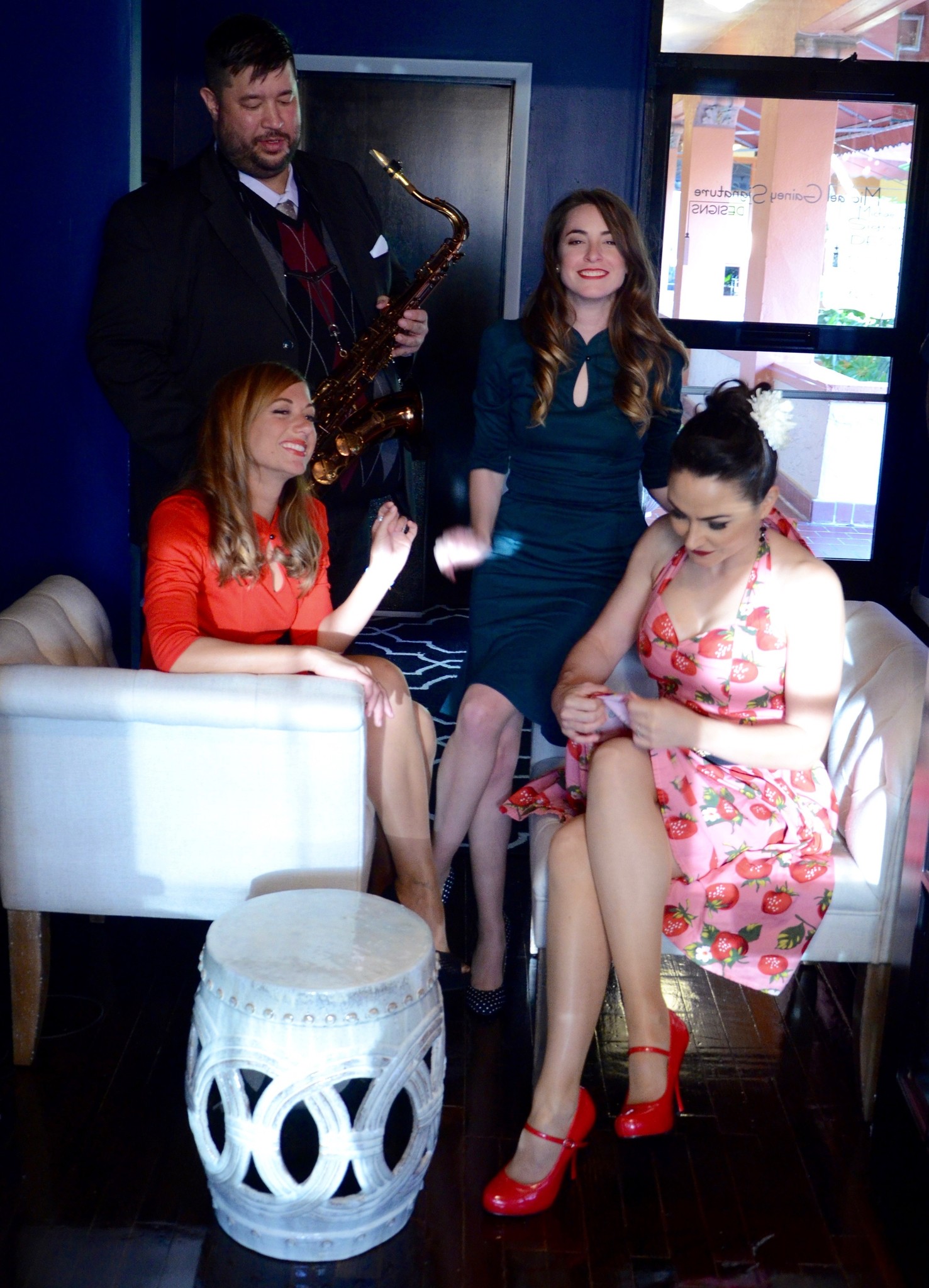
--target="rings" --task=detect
[377,516,383,521]
[402,528,405,530]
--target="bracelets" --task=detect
[365,567,395,590]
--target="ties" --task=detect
[280,200,296,220]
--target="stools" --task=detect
[183,887,449,1263]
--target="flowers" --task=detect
[745,387,796,451]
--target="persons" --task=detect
[483,374,846,1220]
[428,189,686,1027]
[91,13,431,672]
[144,359,470,974]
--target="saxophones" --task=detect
[305,145,471,487]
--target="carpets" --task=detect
[352,605,533,850]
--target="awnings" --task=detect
[671,0,929,156]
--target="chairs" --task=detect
[528,594,929,1120]
[0,570,379,1067]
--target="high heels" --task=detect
[466,916,509,1016]
[442,867,456,906]
[615,1010,690,1139]
[484,1085,596,1215]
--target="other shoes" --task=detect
[438,950,459,996]
